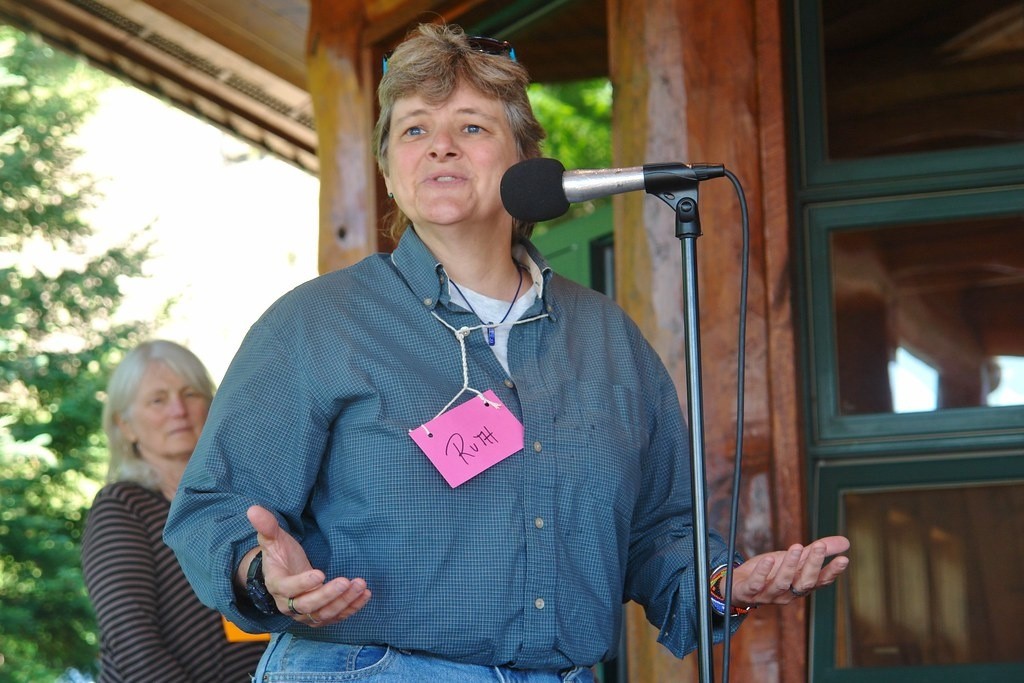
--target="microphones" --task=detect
[499,156,725,224]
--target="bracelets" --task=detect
[707,562,755,618]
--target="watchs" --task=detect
[246,550,279,618]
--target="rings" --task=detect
[288,596,303,615]
[789,584,808,596]
[307,613,319,623]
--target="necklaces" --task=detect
[449,259,523,346]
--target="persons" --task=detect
[161,26,851,683]
[79,339,269,683]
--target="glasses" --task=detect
[382,35,517,77]
